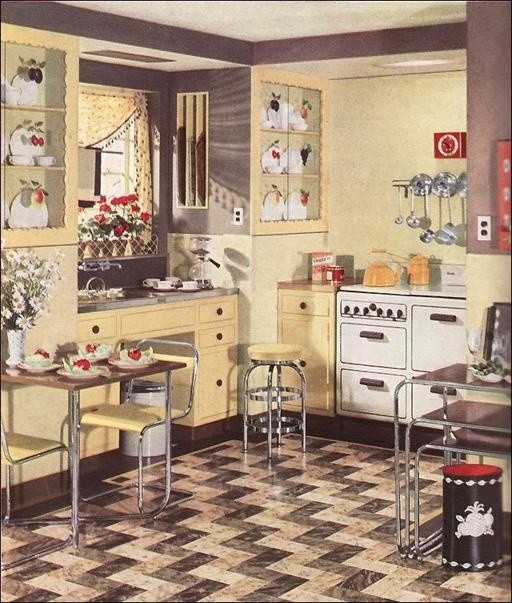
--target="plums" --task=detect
[28,68,42,84]
[270,100,280,112]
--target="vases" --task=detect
[3,327,25,370]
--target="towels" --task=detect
[11,341,154,378]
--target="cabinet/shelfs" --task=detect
[168,294,240,440]
[74,312,121,460]
[275,280,340,417]
[120,304,193,333]
[166,67,329,236]
[1,22,80,247]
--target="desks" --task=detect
[389,361,512,563]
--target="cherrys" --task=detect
[272,151,280,159]
[31,135,44,146]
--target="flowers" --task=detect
[78,191,151,256]
[1,238,62,330]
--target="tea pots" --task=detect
[363,248,404,286]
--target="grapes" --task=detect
[300,149,308,166]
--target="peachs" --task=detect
[273,190,280,206]
[30,189,46,208]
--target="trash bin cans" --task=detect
[120,380,168,458]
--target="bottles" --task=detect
[408,252,429,285]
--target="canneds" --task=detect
[324,264,344,284]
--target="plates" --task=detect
[67,353,108,362]
[261,101,308,222]
[107,358,159,368]
[7,71,49,229]
[17,361,62,373]
[56,368,100,379]
[472,372,503,383]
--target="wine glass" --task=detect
[465,326,481,371]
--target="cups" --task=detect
[79,288,122,301]
[141,275,201,292]
[36,156,56,166]
[325,266,344,283]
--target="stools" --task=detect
[242,341,310,464]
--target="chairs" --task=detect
[77,337,201,519]
[0,407,79,572]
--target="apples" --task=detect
[299,194,308,207]
[74,358,90,370]
[86,344,96,353]
[128,348,141,360]
[33,349,49,359]
[299,107,308,118]
